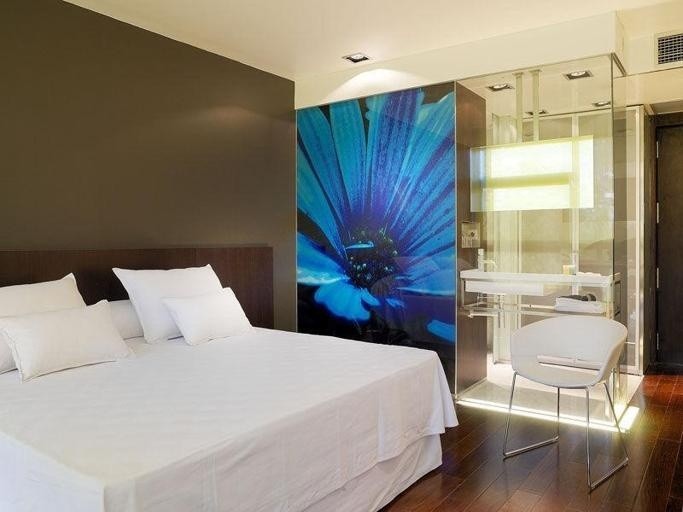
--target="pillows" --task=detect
[0,269,87,377]
[111,264,222,344]
[0,298,131,382]
[108,300,145,342]
[158,286,253,346]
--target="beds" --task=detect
[0,245,460,512]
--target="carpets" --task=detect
[462,381,615,424]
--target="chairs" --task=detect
[497,315,631,494]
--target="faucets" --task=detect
[477,248,499,272]
